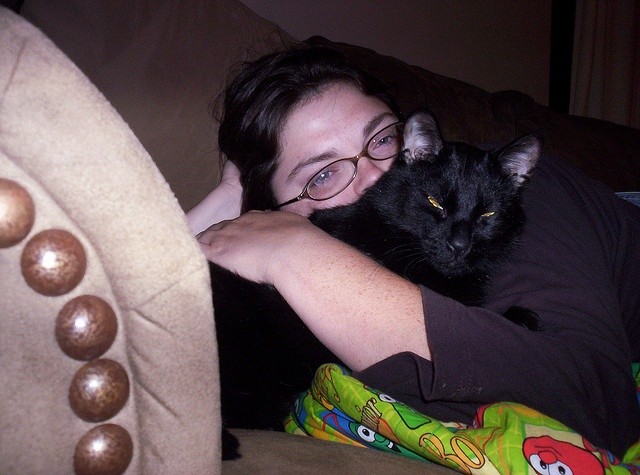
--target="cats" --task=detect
[207,111,542,461]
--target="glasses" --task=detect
[276,120,409,208]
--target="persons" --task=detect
[183,45,640,463]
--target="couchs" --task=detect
[2,2,639,473]
